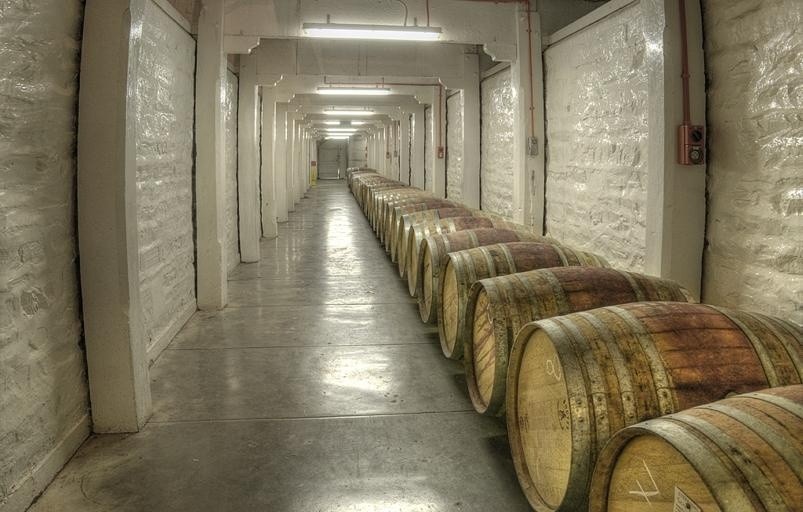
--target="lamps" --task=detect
[320,119,365,140]
[321,105,378,116]
[300,22,443,41]
[316,85,392,97]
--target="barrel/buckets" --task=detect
[390,201,457,263]
[436,242,615,361]
[417,224,564,327]
[386,197,444,254]
[399,207,488,280]
[346,166,433,243]
[504,301,803,512]
[464,266,699,415]
[405,213,511,298]
[587,384,801,510]
